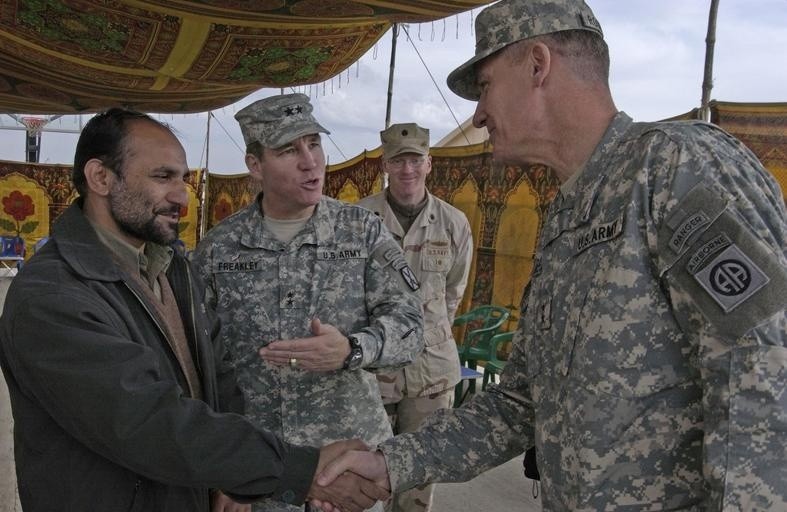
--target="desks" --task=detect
[0,256,24,277]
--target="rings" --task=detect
[288,357,297,367]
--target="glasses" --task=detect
[385,158,425,167]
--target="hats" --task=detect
[380,123,429,159]
[234,93,331,150]
[447,0,603,100]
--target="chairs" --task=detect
[0,237,51,270]
[453,305,515,407]
[173,239,195,259]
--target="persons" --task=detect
[186,92,425,510]
[0,107,391,511]
[349,122,475,511]
[299,1,784,510]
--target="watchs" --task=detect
[343,335,363,372]
